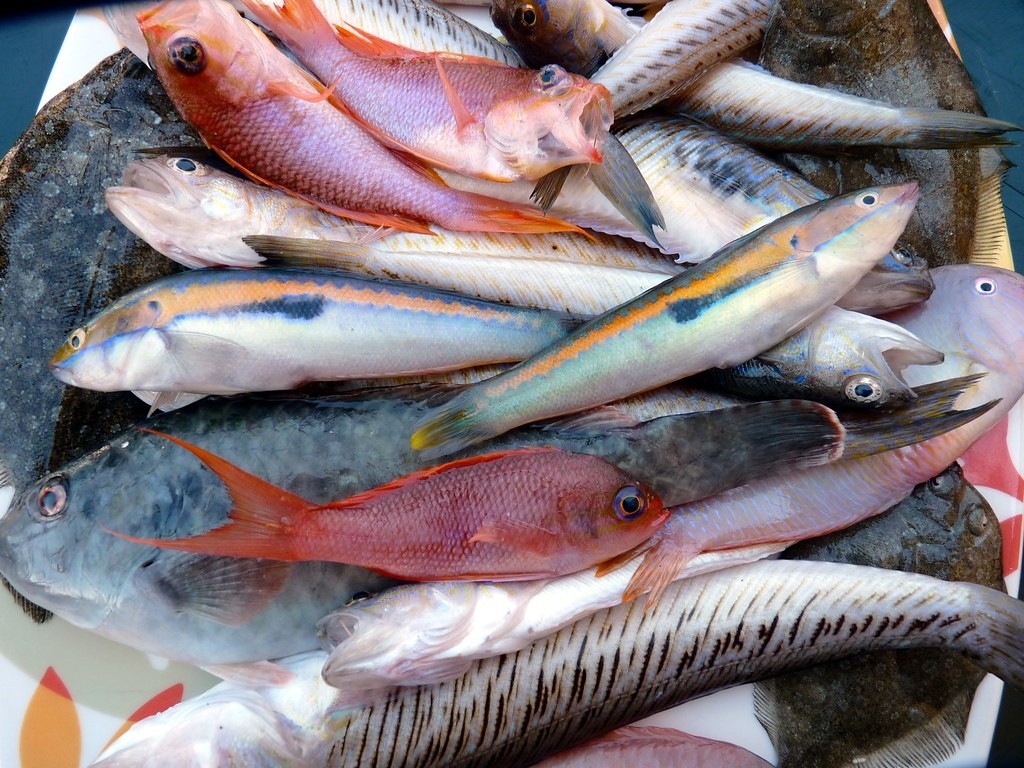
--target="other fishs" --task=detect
[0,2,1024,768]
